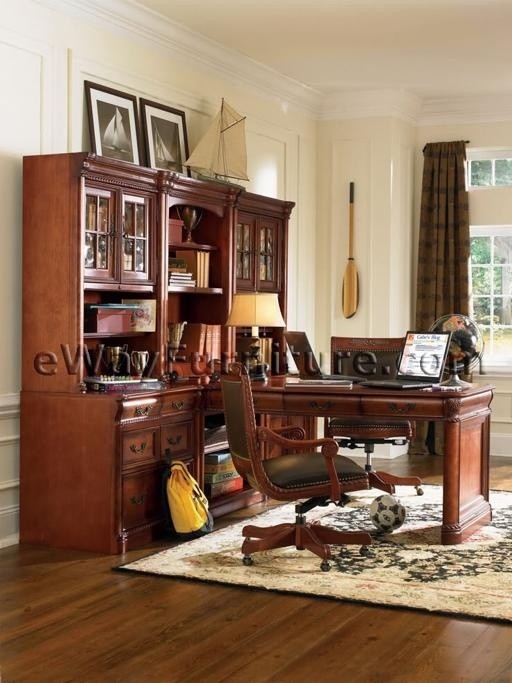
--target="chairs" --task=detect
[212,360,374,572]
[327,334,427,495]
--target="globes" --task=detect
[428,313,485,391]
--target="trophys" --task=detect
[176,204,204,244]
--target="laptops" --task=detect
[359,330,453,389]
[283,331,368,384]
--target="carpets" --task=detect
[113,484,512,623]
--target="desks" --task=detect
[202,375,498,542]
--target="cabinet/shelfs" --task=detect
[19,375,317,553]
[22,149,295,393]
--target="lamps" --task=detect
[226,290,286,375]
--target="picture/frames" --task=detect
[138,93,193,178]
[82,79,146,167]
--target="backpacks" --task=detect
[161,461,214,537]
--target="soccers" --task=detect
[369,495,406,532]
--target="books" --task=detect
[168,257,196,287]
[205,452,243,498]
[177,250,209,287]
[240,337,272,371]
[285,377,351,388]
[123,300,156,332]
[183,322,238,374]
[91,302,143,309]
